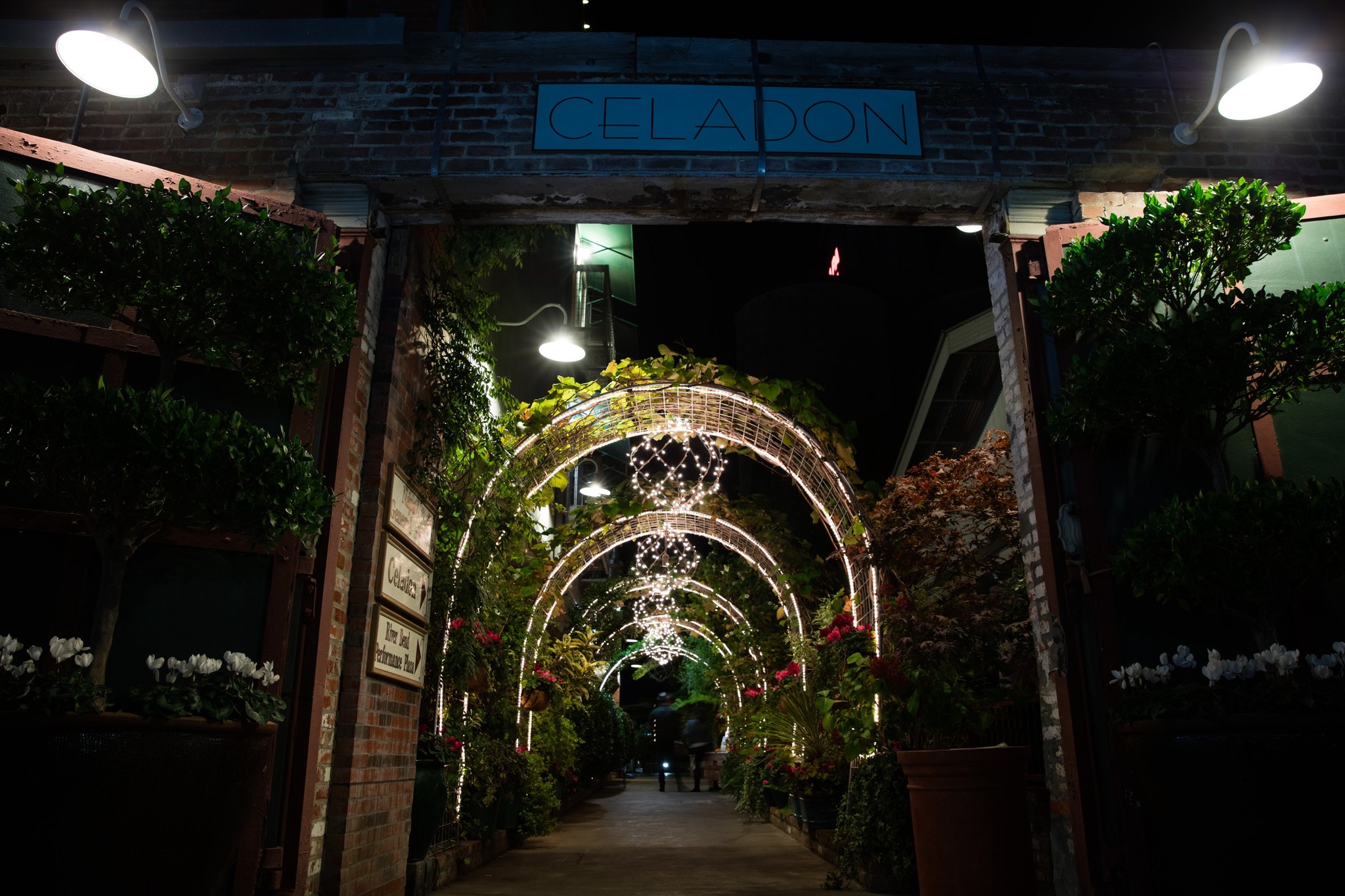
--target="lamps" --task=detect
[622,436,730,659]
[567,455,611,496]
[55,2,202,133]
[489,297,591,368]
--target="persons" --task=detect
[682,711,722,794]
[648,691,692,791]
